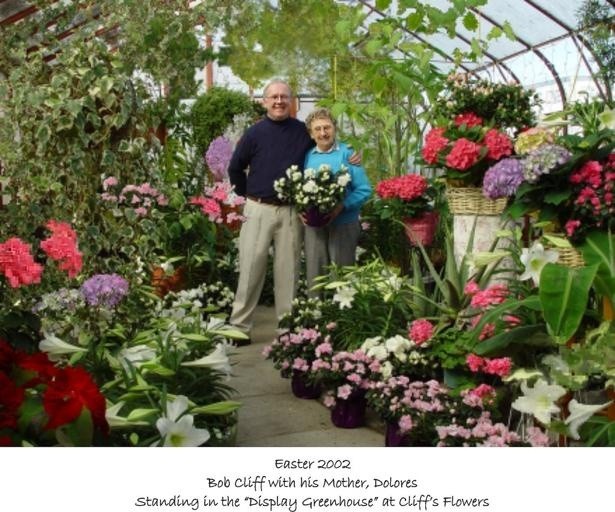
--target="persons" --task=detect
[222,76,363,348]
[297,107,374,306]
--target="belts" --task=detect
[245,195,280,206]
